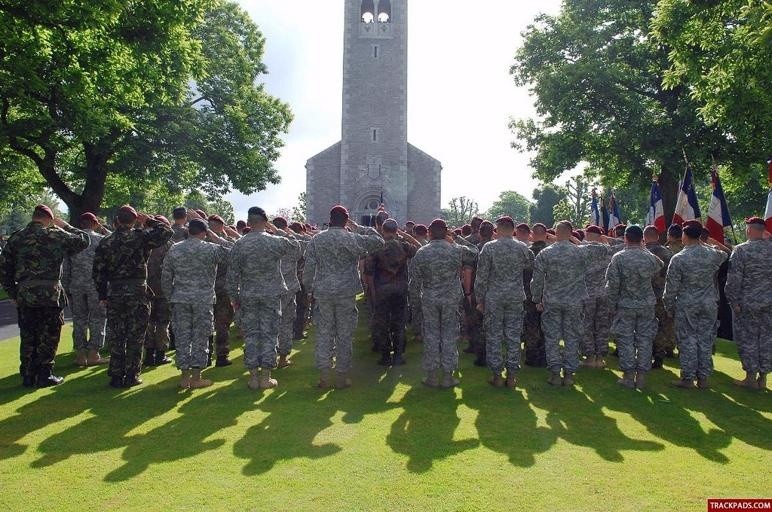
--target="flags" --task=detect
[762,159,772,241]
[607,192,622,232]
[671,163,703,226]
[590,192,600,226]
[645,182,667,235]
[598,197,609,229]
[704,165,732,245]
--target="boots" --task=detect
[637,371,645,388]
[319,368,331,387]
[261,367,277,389]
[336,371,352,389]
[179,370,191,389]
[377,353,392,365]
[88,351,108,366]
[248,367,259,389]
[74,351,87,366]
[735,371,759,389]
[192,369,211,389]
[758,373,767,390]
[393,352,404,365]
[618,370,635,388]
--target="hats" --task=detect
[461,225,471,231]
[414,224,428,231]
[208,214,225,224]
[291,221,302,230]
[454,229,462,233]
[330,206,349,216]
[154,214,170,225]
[120,205,138,217]
[273,217,288,227]
[195,210,209,220]
[764,217,772,234]
[572,231,583,242]
[249,207,267,220]
[585,226,601,233]
[682,220,701,227]
[430,219,447,228]
[174,208,186,213]
[745,217,766,224]
[479,220,492,228]
[578,230,585,238]
[406,221,414,226]
[237,220,245,227]
[376,212,389,219]
[80,213,98,224]
[35,204,53,220]
[190,218,208,231]
[518,223,530,232]
[612,224,627,232]
[496,216,514,227]
[546,230,556,235]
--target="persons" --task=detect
[235,220,247,236]
[603,225,666,390]
[567,231,582,246]
[301,204,386,391]
[223,206,303,391]
[607,229,616,238]
[723,237,732,251]
[578,224,626,369]
[160,217,236,390]
[463,216,485,354]
[363,217,424,367]
[401,221,415,236]
[522,222,556,369]
[723,216,772,391]
[407,217,480,388]
[544,229,556,244]
[170,206,210,244]
[358,211,412,352]
[462,219,498,368]
[91,204,175,388]
[303,223,313,237]
[514,223,534,343]
[290,221,316,340]
[699,226,732,375]
[61,211,114,367]
[576,228,585,239]
[661,218,729,390]
[222,226,238,240]
[663,223,686,359]
[643,224,674,369]
[474,215,536,389]
[143,214,178,368]
[270,216,312,367]
[204,213,244,367]
[0,202,92,388]
[449,228,463,242]
[530,220,608,388]
[460,224,472,238]
[407,223,429,344]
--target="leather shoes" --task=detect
[143,349,155,368]
[156,351,173,366]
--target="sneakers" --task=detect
[421,376,439,388]
[563,376,575,385]
[609,347,618,356]
[39,374,63,387]
[474,357,487,367]
[462,344,477,354]
[652,358,662,368]
[539,357,546,367]
[524,359,537,368]
[111,376,124,388]
[294,331,309,340]
[216,356,231,367]
[23,376,37,388]
[673,379,694,387]
[666,349,674,357]
[549,375,562,387]
[598,356,607,367]
[207,357,212,367]
[697,377,708,388]
[506,376,519,389]
[442,376,461,388]
[278,354,293,367]
[583,356,596,366]
[125,376,142,388]
[494,375,505,388]
